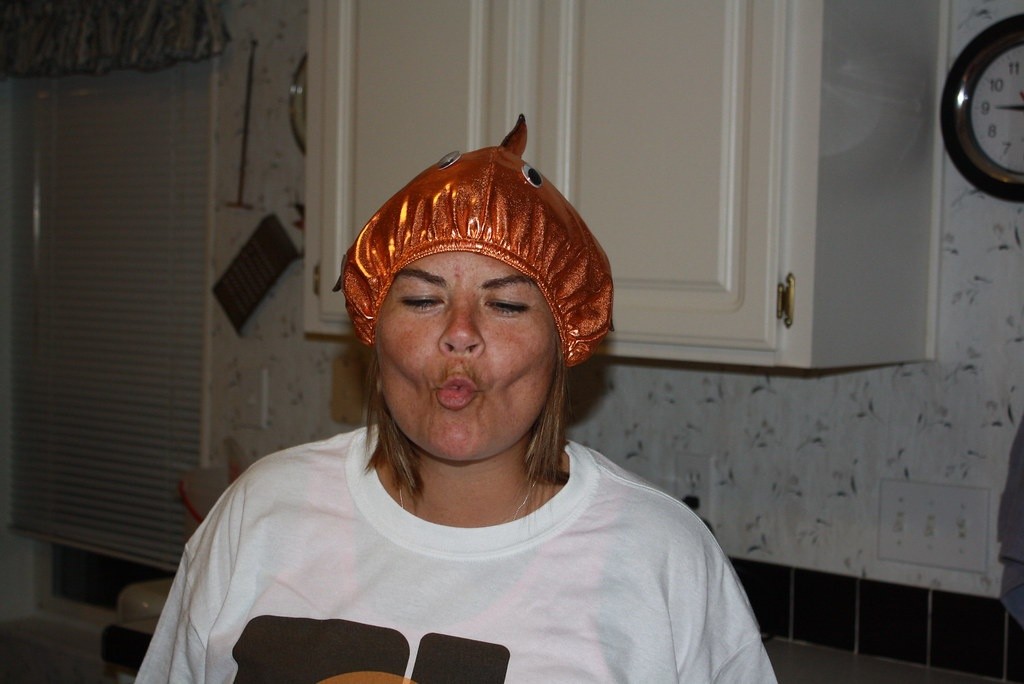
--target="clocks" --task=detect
[939,10,1024,206]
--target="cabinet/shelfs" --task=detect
[302,3,943,372]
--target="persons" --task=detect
[131,113,785,684]
[997,403,1024,642]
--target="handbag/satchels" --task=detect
[211,214,303,336]
